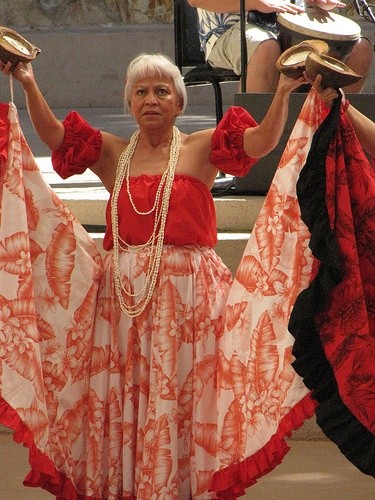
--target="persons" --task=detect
[302,70,375,166]
[187,0,373,94]
[0,53,335,500]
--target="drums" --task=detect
[277,7,361,64]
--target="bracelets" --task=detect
[344,99,350,111]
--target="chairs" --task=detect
[173,0,242,179]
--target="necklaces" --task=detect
[109,124,182,319]
